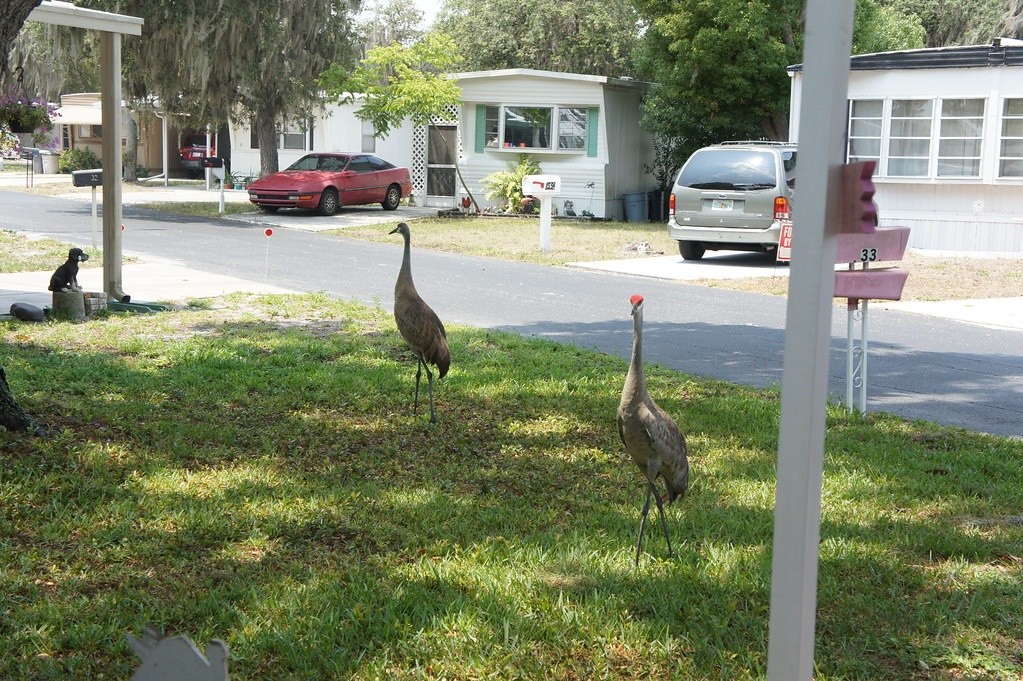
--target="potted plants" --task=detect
[224,168,253,190]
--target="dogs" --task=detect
[48,247,90,292]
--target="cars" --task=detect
[248,151,410,217]
[667,140,800,265]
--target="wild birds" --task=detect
[615,294,689,571]
[388,222,450,425]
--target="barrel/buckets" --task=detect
[623,192,649,223]
[650,191,669,222]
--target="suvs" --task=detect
[178,128,231,177]
